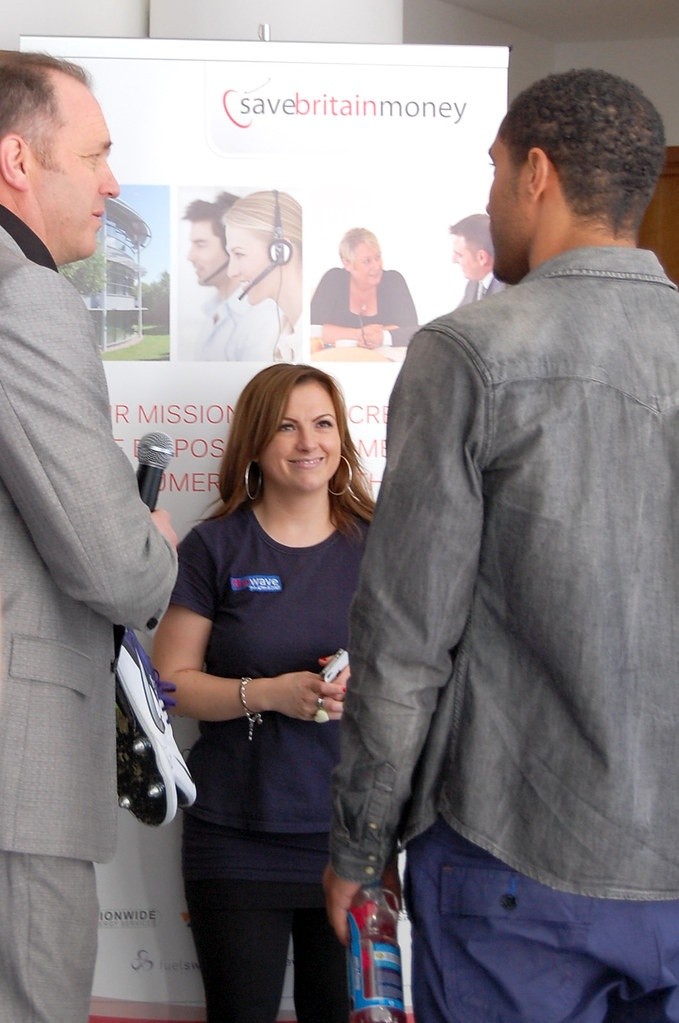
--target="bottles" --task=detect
[345,877,408,1023]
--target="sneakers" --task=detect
[110,621,196,827]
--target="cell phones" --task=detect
[321,649,349,683]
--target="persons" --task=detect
[0,50,183,1023]
[319,66,679,1023]
[448,214,508,308]
[176,191,302,363]
[311,228,418,351]
[155,364,404,1022]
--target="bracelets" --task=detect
[240,678,263,741]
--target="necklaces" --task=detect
[362,305,367,310]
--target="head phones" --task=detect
[268,190,293,264]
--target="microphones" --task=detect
[238,262,279,301]
[358,305,369,346]
[204,261,229,282]
[136,433,175,512]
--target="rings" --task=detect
[313,707,329,723]
[317,696,324,708]
